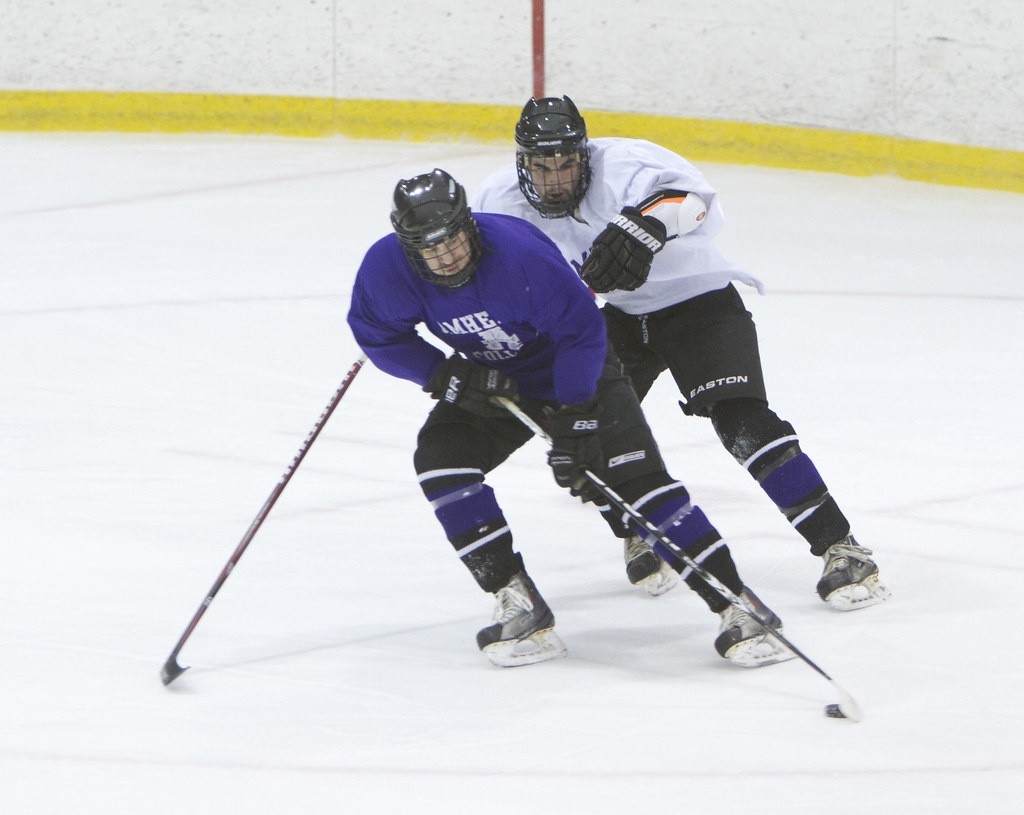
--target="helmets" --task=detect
[390,168,482,291]
[515,95,592,219]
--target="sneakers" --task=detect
[477,552,568,667]
[624,535,679,596]
[714,585,804,668]
[816,535,892,611]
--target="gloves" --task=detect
[547,395,605,504]
[423,351,520,417]
[581,206,666,293]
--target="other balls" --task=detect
[825,702,847,719]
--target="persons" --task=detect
[345,168,799,668]
[471,95,894,612]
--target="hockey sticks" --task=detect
[158,345,369,684]
[494,398,869,725]
